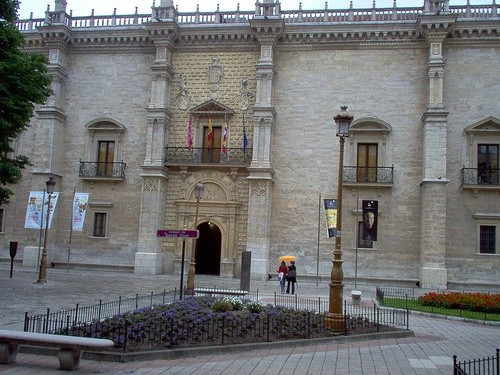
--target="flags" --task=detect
[186,116,193,150]
[207,118,213,148]
[72,193,90,231]
[243,125,248,148]
[24,191,59,229]
[221,122,227,155]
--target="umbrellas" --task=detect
[278,255,296,264]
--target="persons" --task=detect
[286,261,296,294]
[276,261,287,294]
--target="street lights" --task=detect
[36,177,56,283]
[182,180,205,295]
[323,105,355,333]
